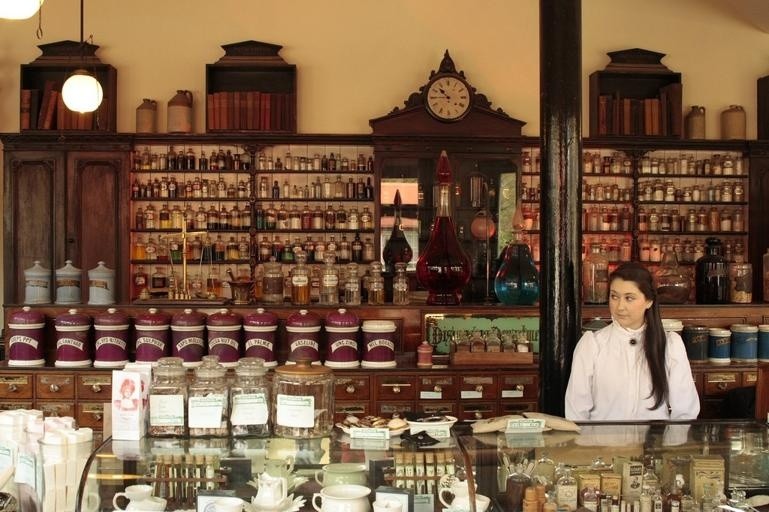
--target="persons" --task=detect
[565,262,700,448]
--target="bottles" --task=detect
[256,273,264,302]
[682,238,693,262]
[650,239,662,263]
[151,453,215,503]
[486,331,502,352]
[383,189,413,303]
[638,235,651,261]
[620,239,632,262]
[653,247,690,304]
[368,263,384,305]
[455,331,470,354]
[310,267,319,302]
[763,248,769,305]
[472,331,486,353]
[673,240,683,264]
[338,268,345,300]
[515,334,532,352]
[263,256,285,304]
[583,244,609,305]
[132,234,251,306]
[726,239,732,263]
[503,451,757,512]
[394,451,457,494]
[345,264,360,305]
[291,252,310,304]
[695,238,726,304]
[320,253,341,304]
[522,146,748,234]
[283,270,293,299]
[259,235,374,260]
[609,238,621,261]
[495,199,542,306]
[601,239,608,261]
[392,263,409,304]
[502,334,516,352]
[729,256,751,304]
[416,150,474,306]
[735,239,744,262]
[694,240,704,262]
[583,240,589,259]
[130,142,373,231]
[362,271,370,302]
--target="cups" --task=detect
[216,498,244,511]
[311,484,371,512]
[313,463,366,486]
[373,500,402,512]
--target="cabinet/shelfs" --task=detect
[582,315,767,403]
[205,38,298,136]
[581,144,753,306]
[32,139,130,306]
[130,140,375,307]
[332,372,540,430]
[369,50,527,302]
[0,372,109,431]
[586,48,683,140]
[19,39,117,132]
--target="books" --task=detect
[208,90,293,131]
[598,83,682,137]
[21,81,110,132]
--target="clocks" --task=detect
[422,70,472,121]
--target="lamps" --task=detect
[62,0,103,113]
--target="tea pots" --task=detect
[112,485,167,511]
[439,483,491,512]
[266,455,294,485]
[253,472,286,507]
